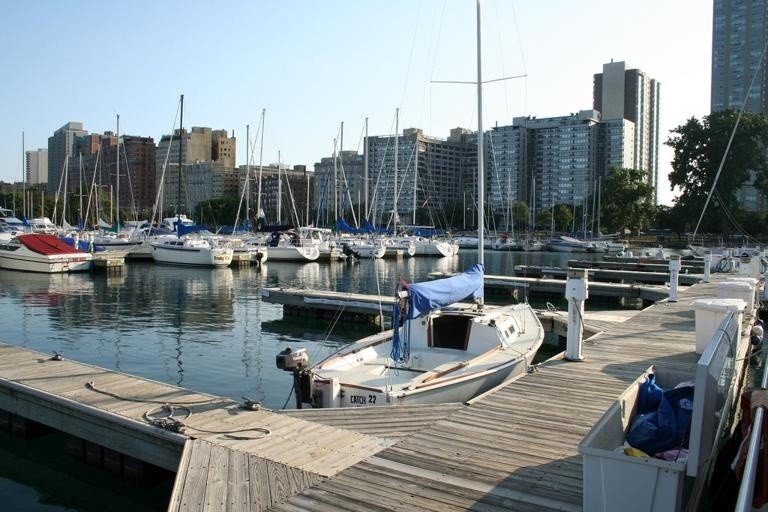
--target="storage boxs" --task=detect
[576,312,739,512]
[692,276,757,356]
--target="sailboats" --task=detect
[274,1,545,408]
[687,42,768,258]
[0,94,630,274]
